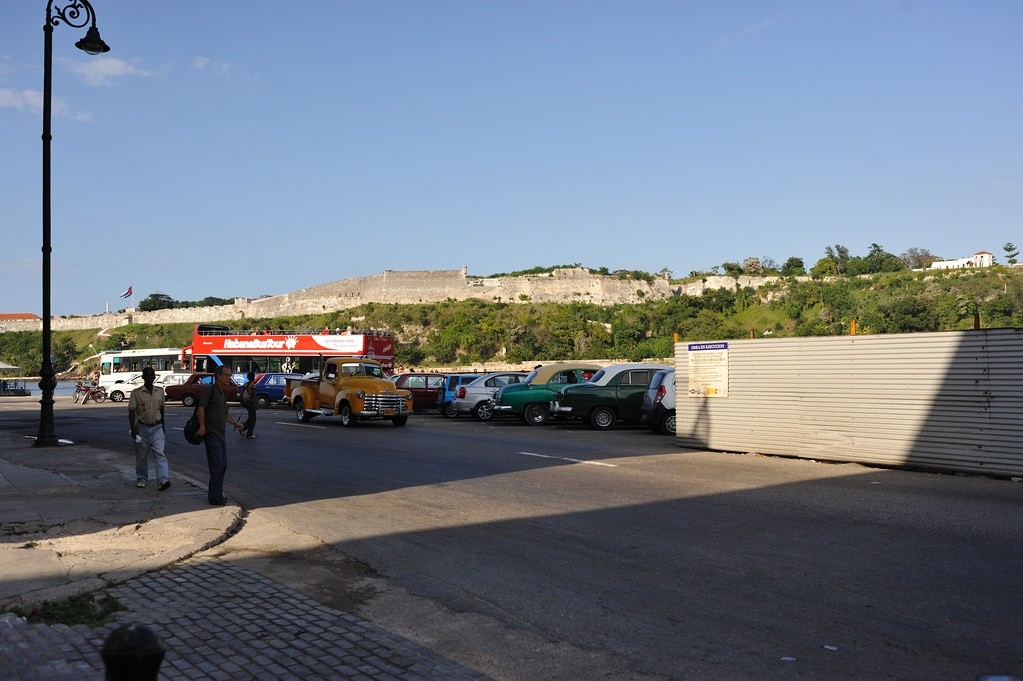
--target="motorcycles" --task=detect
[71,382,107,404]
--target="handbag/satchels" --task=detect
[184,414,205,445]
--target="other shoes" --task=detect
[240,431,245,437]
[247,434,257,438]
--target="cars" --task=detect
[550,363,671,429]
[165,374,243,407]
[488,363,605,426]
[641,369,676,435]
[451,372,528,423]
[237,373,304,408]
[436,374,507,418]
[389,374,446,413]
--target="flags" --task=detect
[120,286,133,300]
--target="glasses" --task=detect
[222,374,232,376]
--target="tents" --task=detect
[0,361,22,377]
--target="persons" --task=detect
[239,372,260,439]
[195,365,244,504]
[0,379,18,392]
[320,325,361,335]
[255,325,284,335]
[581,370,592,382]
[128,367,171,491]
[93,372,99,388]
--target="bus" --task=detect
[97,346,205,387]
[182,324,395,383]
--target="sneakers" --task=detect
[158,480,171,491]
[137,479,147,487]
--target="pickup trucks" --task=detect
[281,356,413,427]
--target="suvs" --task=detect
[104,372,176,400]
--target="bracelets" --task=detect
[233,421,238,425]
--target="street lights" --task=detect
[32,0,113,446]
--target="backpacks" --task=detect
[240,387,250,405]
